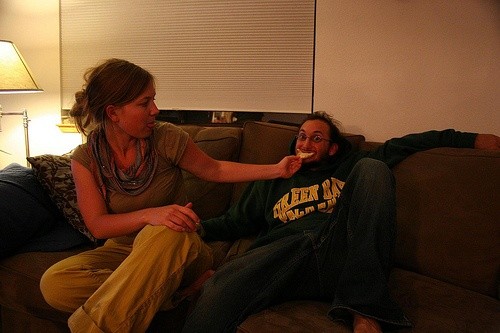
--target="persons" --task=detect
[152,110,499,332]
[35,58,304,333]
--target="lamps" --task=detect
[0,39,45,169]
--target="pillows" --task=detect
[25,152,105,245]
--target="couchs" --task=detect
[0,121,498,333]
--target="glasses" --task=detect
[295,133,331,143]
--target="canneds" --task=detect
[212,111,233,123]
